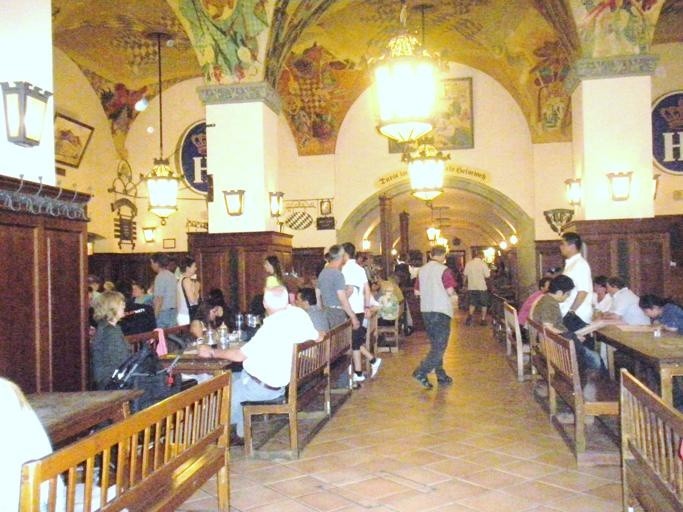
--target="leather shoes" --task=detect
[229,431,246,446]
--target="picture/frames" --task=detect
[53,109,95,170]
[387,77,475,153]
[162,238,176,249]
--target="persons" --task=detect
[462,251,490,326]
[600,277,650,326]
[411,245,456,389]
[510,276,552,346]
[199,285,325,449]
[638,295,682,333]
[88,251,230,467]
[317,242,414,388]
[529,274,598,388]
[0,377,130,511]
[264,255,330,333]
[592,275,607,303]
[556,230,595,387]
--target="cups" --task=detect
[204,311,257,350]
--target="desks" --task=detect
[20,387,144,449]
[588,317,681,407]
[155,327,262,397]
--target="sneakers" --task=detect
[463,316,470,325]
[480,318,488,325]
[438,374,452,383]
[412,369,433,387]
[370,359,381,377]
[352,371,365,382]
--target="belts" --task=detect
[328,305,343,309]
[244,369,283,390]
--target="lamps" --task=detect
[137,30,186,228]
[542,207,576,236]
[399,133,454,204]
[141,226,157,244]
[222,188,246,218]
[607,171,634,202]
[0,80,52,148]
[371,0,447,144]
[652,172,661,202]
[563,177,582,208]
[267,189,285,218]
[423,206,452,264]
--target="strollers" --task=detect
[57,332,197,489]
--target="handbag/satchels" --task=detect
[190,302,205,323]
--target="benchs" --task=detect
[455,266,680,510]
[0,267,414,511]
